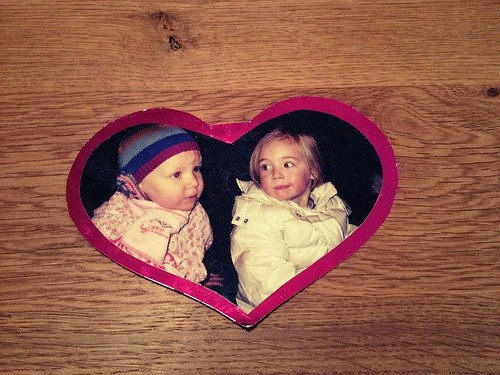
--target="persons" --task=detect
[229,125,360,315]
[90,123,225,287]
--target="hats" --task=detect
[117,123,201,201]
